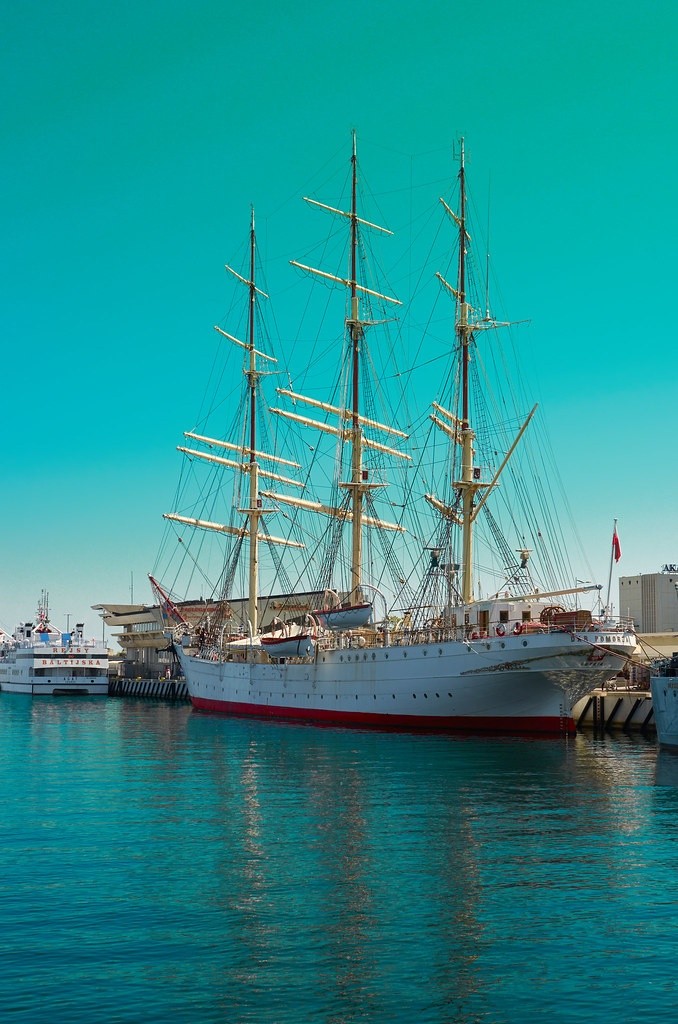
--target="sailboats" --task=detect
[147,130,636,737]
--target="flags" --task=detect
[613,531,621,563]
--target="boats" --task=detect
[0,588,109,694]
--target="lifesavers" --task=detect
[495,623,507,637]
[512,621,522,635]
